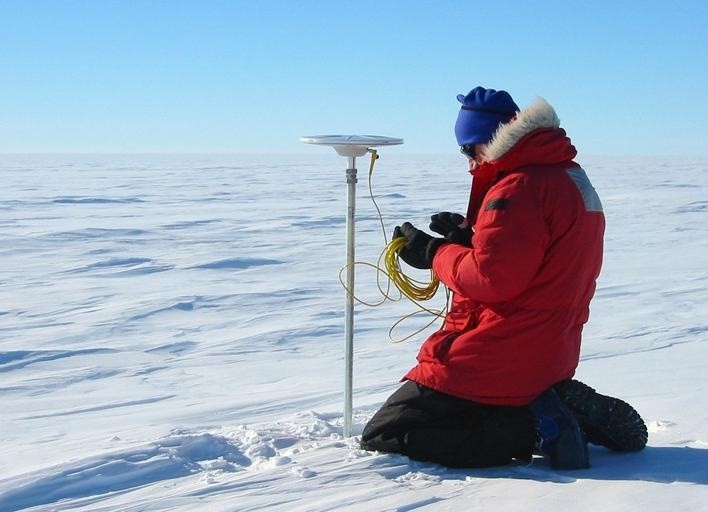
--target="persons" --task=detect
[360,86,647,471]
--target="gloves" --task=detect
[392,222,446,269]
[429,212,473,247]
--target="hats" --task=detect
[455,87,520,146]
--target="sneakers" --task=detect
[531,387,588,470]
[552,380,648,451]
